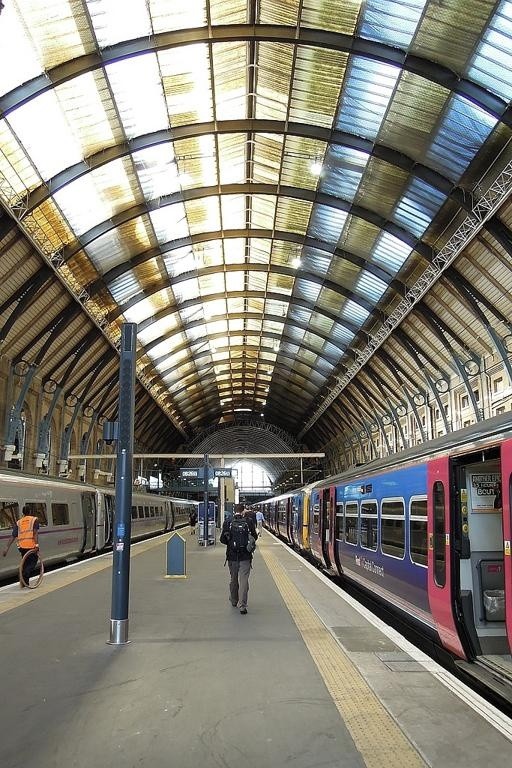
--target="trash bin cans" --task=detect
[163,531,188,579]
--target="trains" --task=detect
[247,412,511,709]
[0,468,198,583]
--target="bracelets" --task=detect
[34,543,39,548]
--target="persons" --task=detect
[2,504,40,589]
[219,503,258,614]
[187,508,198,535]
[223,505,266,538]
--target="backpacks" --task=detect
[227,518,251,551]
